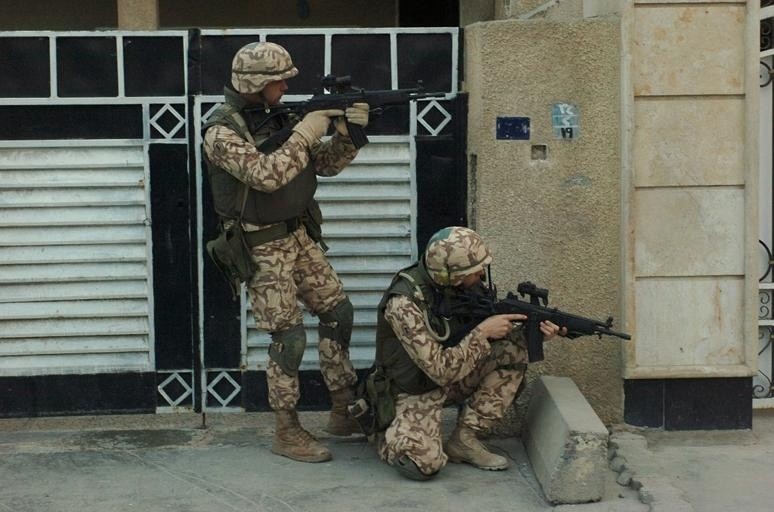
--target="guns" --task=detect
[432,279,632,362]
[241,72,445,148]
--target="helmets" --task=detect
[231,41,299,95]
[425,225,493,285]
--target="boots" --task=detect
[445,426,508,471]
[325,388,362,437]
[269,409,331,463]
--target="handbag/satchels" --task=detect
[205,226,259,288]
[354,372,396,433]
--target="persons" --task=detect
[366,225,567,482]
[202,42,370,464]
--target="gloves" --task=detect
[333,103,370,137]
[293,108,345,149]
